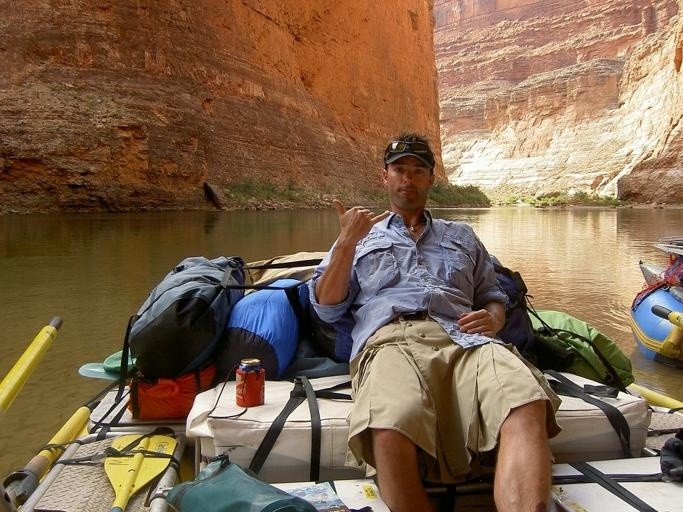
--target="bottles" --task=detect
[235,358,265,407]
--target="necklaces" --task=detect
[401,217,423,233]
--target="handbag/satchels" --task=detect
[308,254,535,357]
[526,307,635,395]
[127,257,309,418]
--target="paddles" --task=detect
[103,432,178,512]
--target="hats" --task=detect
[384,139,435,168]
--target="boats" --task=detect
[628,239,681,369]
[16,313,681,511]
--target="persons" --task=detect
[307,129,556,512]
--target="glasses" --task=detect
[385,140,431,155]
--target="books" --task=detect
[280,481,349,512]
